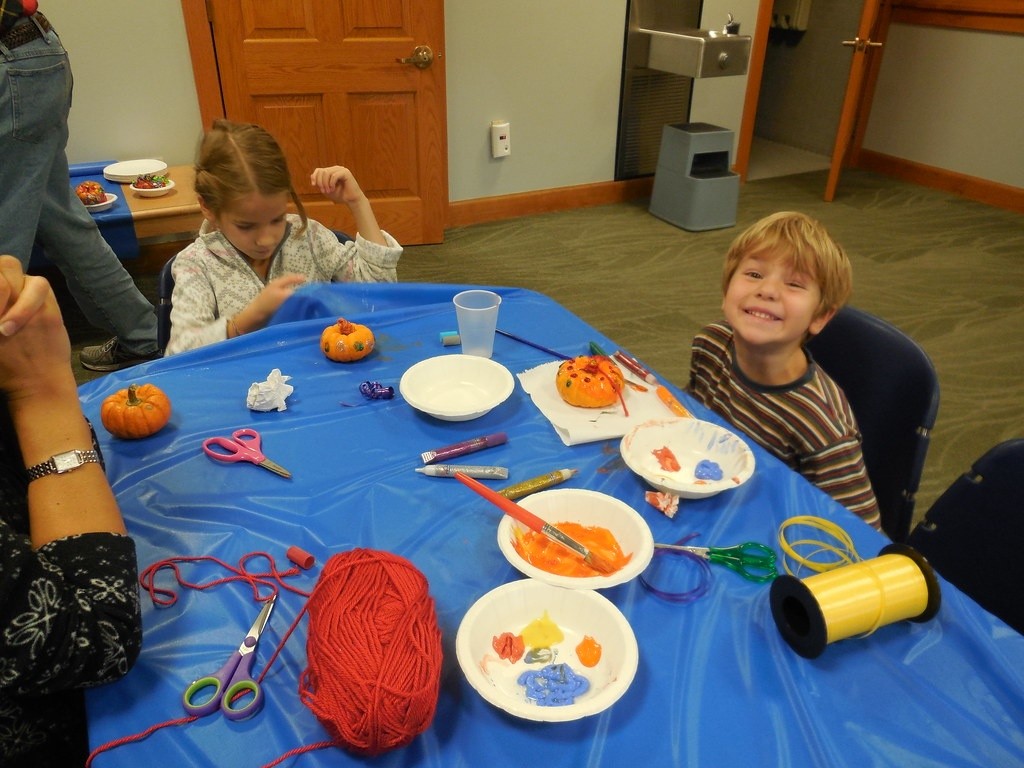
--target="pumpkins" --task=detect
[76,181,107,205]
[557,353,624,407]
[101,383,171,439]
[320,318,375,362]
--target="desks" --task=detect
[75,281,1024,768]
[120,162,205,238]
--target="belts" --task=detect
[0,13,49,55]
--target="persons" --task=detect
[0,254,142,768]
[0,0,162,368]
[683,213,892,542]
[162,120,404,360]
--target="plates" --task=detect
[495,488,654,590]
[620,417,756,500]
[129,180,175,198]
[399,353,515,421]
[455,577,639,723]
[84,192,118,211]
[103,158,169,183]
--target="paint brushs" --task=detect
[495,328,647,393]
[454,471,618,575]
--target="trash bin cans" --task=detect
[648,122,740,231]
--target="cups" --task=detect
[452,290,502,359]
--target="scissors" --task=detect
[182,594,278,721]
[654,542,777,584]
[202,429,292,479]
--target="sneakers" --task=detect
[79,336,160,371]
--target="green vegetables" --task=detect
[131,174,169,185]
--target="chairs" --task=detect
[157,232,353,361]
[909,438,1024,640]
[806,304,940,545]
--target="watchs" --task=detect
[25,449,101,483]
